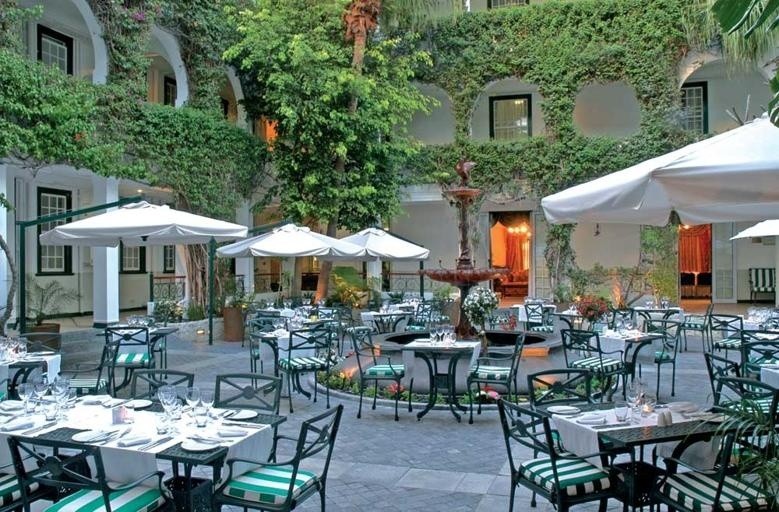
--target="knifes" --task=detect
[139,434,176,453]
[567,412,586,419]
[23,421,57,436]
[591,423,629,430]
[87,428,121,442]
[112,396,134,408]
[223,408,241,418]
[558,406,583,412]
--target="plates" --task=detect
[219,409,259,421]
[124,399,152,410]
[546,404,581,414]
[0,408,26,416]
[667,398,729,425]
[71,430,111,443]
[181,436,221,451]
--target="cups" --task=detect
[629,406,643,425]
[614,400,628,421]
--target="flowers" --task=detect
[575,295,611,334]
[462,284,499,364]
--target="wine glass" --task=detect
[612,315,636,337]
[127,315,150,327]
[566,301,579,314]
[0,335,27,361]
[642,296,671,310]
[747,304,773,323]
[424,324,457,346]
[17,374,72,421]
[270,316,306,335]
[626,376,658,410]
[263,296,328,309]
[288,307,311,319]
[523,295,545,304]
[157,382,216,426]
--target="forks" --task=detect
[107,428,131,445]
[222,422,265,430]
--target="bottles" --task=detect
[657,411,673,429]
[111,408,137,425]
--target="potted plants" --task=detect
[23,274,83,351]
[219,273,258,342]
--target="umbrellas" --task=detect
[729,219,779,241]
[541,108,779,227]
[208,215,367,344]
[20,195,249,334]
[318,225,431,298]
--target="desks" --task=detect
[0,394,287,511]
[536,397,768,512]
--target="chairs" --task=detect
[651,428,778,511]
[1,436,175,510]
[0,298,779,426]
[653,376,778,474]
[749,267,776,305]
[527,368,618,509]
[214,405,342,511]
[499,399,635,510]
